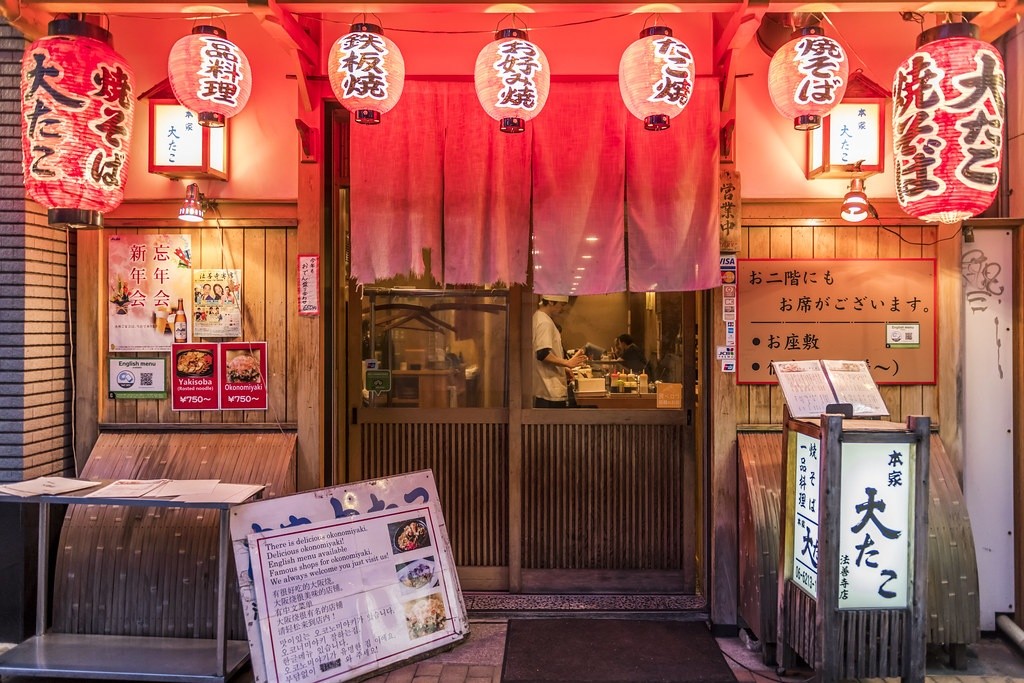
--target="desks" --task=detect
[566,349,657,408]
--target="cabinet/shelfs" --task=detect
[0,481,271,683]
[363,363,467,407]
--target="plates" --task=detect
[177,351,213,376]
[398,559,438,593]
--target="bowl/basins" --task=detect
[394,519,428,553]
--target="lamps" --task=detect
[840,179,868,222]
[178,183,211,223]
[806,68,892,179]
[137,77,232,181]
[776,404,931,683]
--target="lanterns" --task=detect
[620,26,696,131]
[475,29,551,133]
[767,26,850,131]
[327,23,406,126]
[21,19,133,231]
[168,26,252,129]
[891,22,1005,224]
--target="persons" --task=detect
[532,295,588,408]
[619,334,649,376]
[194,284,234,320]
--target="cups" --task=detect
[167,314,176,333]
[155,311,169,334]
[400,361,407,371]
[617,382,624,393]
[605,376,610,385]
[649,384,654,393]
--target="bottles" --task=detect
[628,369,635,382]
[611,369,618,386]
[174,298,187,343]
[639,369,648,394]
[620,369,627,382]
